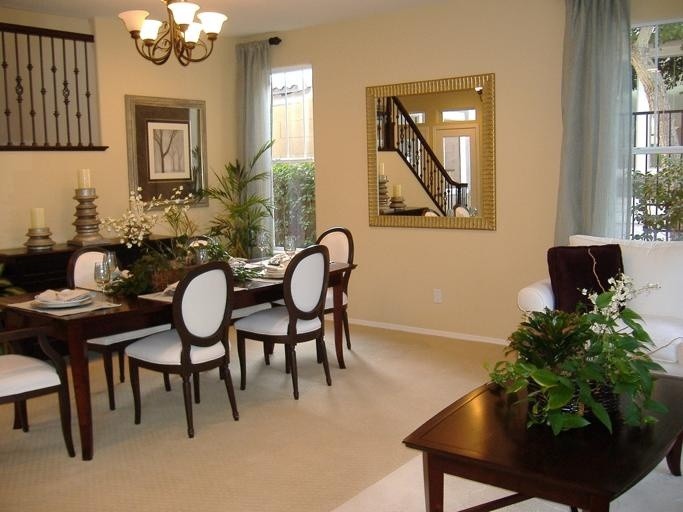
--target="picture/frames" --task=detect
[124,94,210,208]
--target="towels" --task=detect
[34,288,92,301]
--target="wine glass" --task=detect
[94,262,111,307]
[255,231,270,274]
[195,248,209,265]
[283,235,297,264]
[102,252,117,295]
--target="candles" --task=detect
[76,167,92,190]
[392,184,402,196]
[30,206,46,229]
[379,163,385,175]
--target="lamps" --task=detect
[474,87,483,102]
[118,0,228,67]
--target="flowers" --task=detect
[103,186,201,297]
[481,271,673,439]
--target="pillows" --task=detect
[547,244,629,319]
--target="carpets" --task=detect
[327,442,683,512]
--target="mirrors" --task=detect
[366,73,496,230]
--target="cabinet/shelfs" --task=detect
[378,207,428,215]
[0,233,181,361]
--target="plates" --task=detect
[34,290,97,308]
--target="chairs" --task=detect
[126,262,240,437]
[452,202,470,218]
[0,320,76,459]
[266,227,355,369]
[184,235,273,364]
[66,246,172,411]
[233,243,331,399]
[421,208,440,216]
[516,233,683,379]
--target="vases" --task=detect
[136,265,198,292]
[525,382,621,430]
